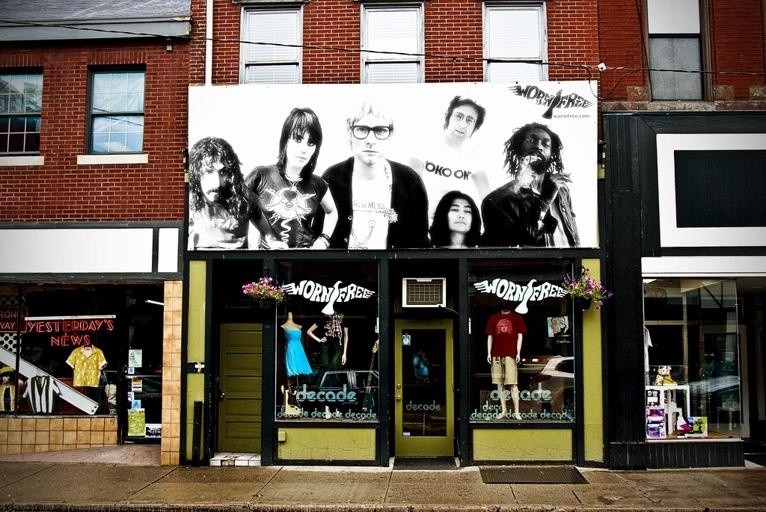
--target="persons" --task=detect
[279,311,313,379]
[244,106,338,252]
[479,122,581,250]
[310,98,429,251]
[305,310,348,377]
[186,136,251,251]
[430,190,481,249]
[408,95,493,246]
[483,305,527,421]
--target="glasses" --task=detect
[349,123,393,141]
[452,113,476,125]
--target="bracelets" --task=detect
[318,232,332,247]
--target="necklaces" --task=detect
[284,172,303,182]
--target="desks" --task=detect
[645,384,691,434]
[716,407,740,430]
